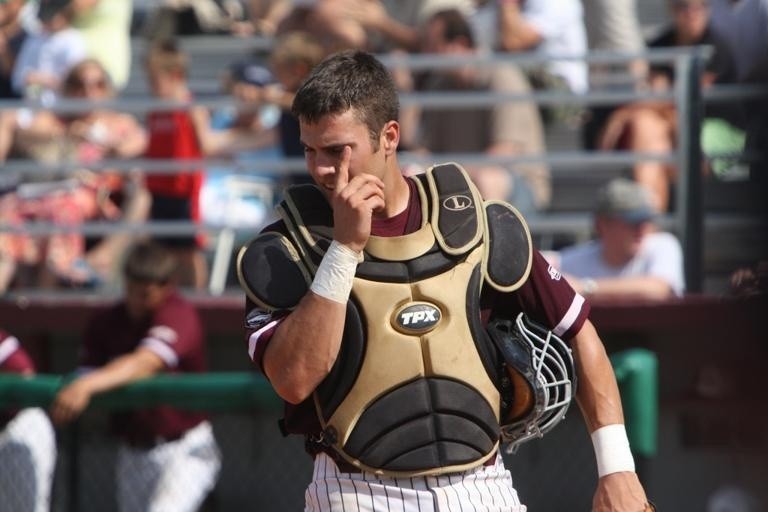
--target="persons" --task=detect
[233,46,656,511]
[47,240,224,511]
[0,0,766,303]
[0,316,67,510]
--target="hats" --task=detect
[123,242,179,284]
[592,176,656,227]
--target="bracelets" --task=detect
[588,422,636,484]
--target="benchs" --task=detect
[123,30,768,297]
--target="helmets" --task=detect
[485,310,578,456]
